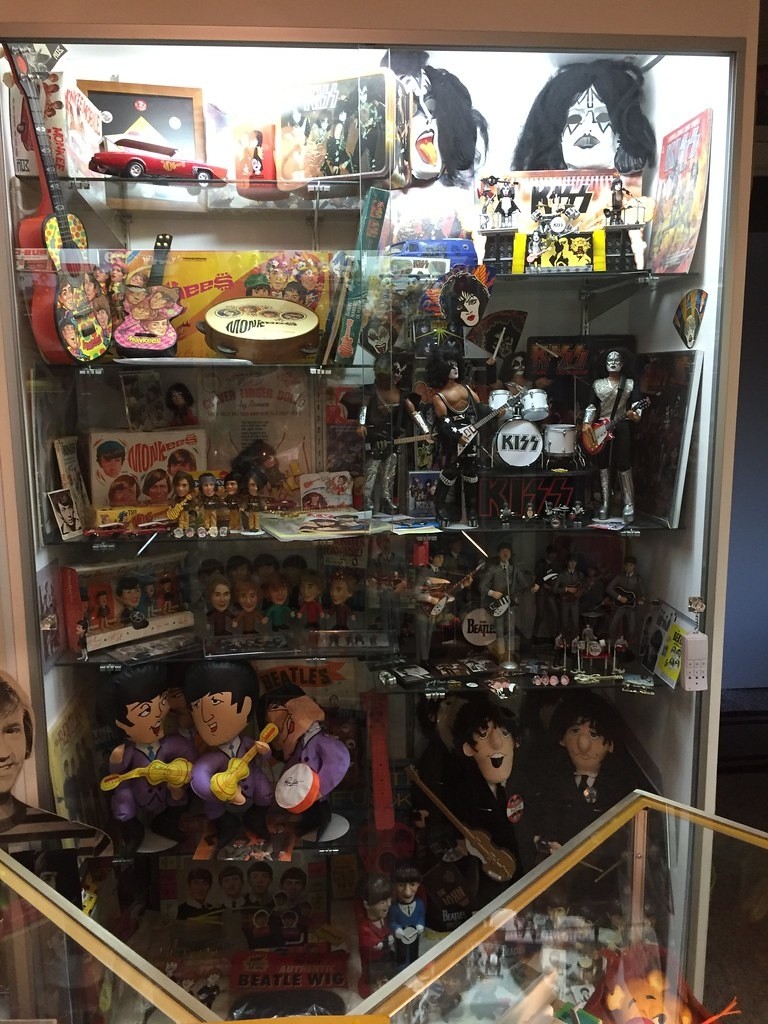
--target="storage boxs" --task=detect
[336,256,452,361]
[126,250,386,366]
[198,369,326,512]
[62,550,191,655]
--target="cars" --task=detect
[89,151,230,189]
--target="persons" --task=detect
[487,176,625,269]
[80,667,360,847]
[57,551,363,649]
[357,349,702,528]
[412,697,640,914]
[0,667,113,863]
[388,542,664,662]
[51,495,82,534]
[173,473,264,536]
[356,865,427,989]
[96,441,195,505]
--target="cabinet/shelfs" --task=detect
[0,0,763,1024]
